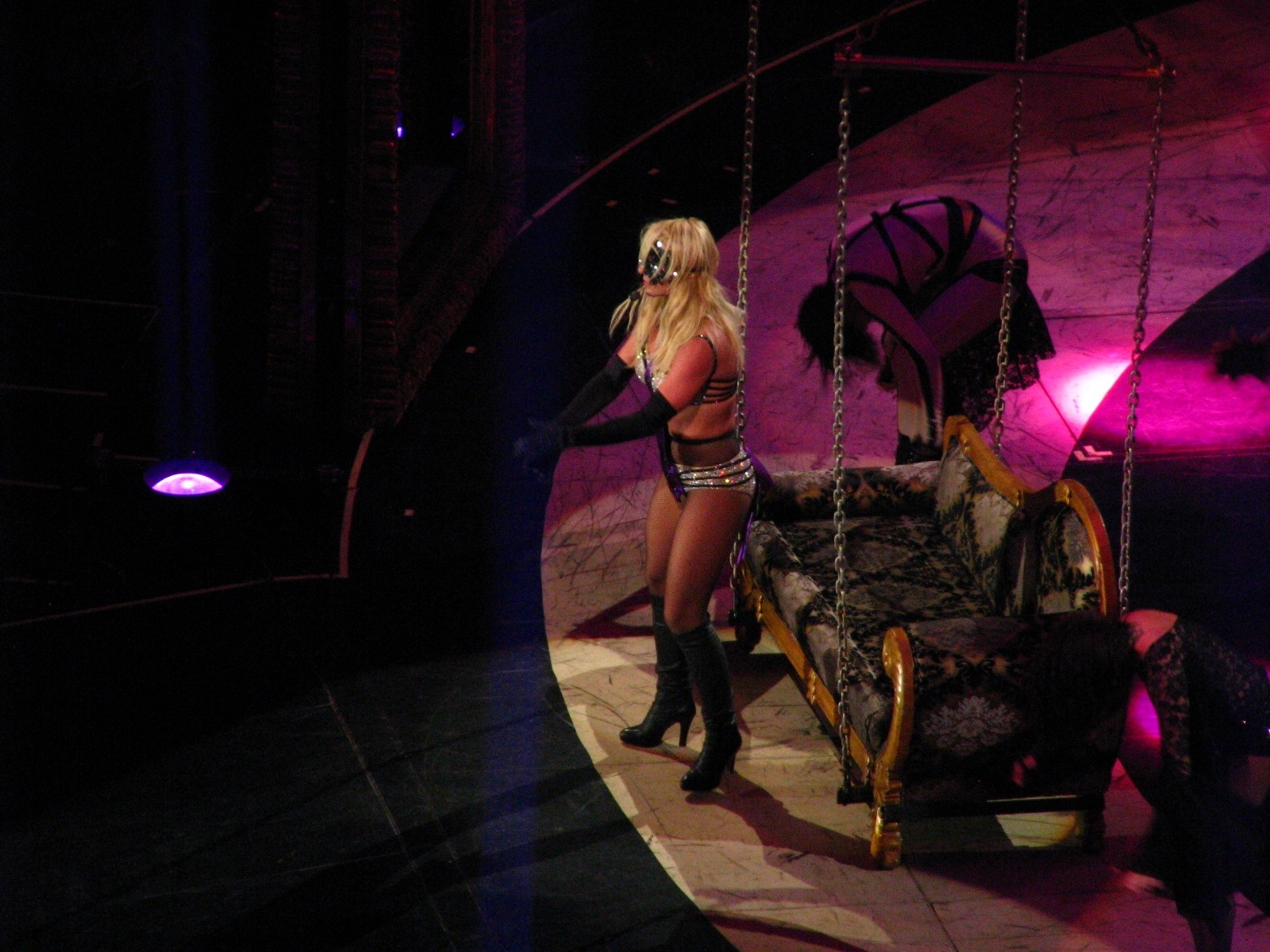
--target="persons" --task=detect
[794,193,1056,466]
[1023,610,1270,952]
[530,216,758,790]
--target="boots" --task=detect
[620,594,697,745]
[670,615,742,792]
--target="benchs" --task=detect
[727,413,1133,868]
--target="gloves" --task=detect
[507,356,677,473]
